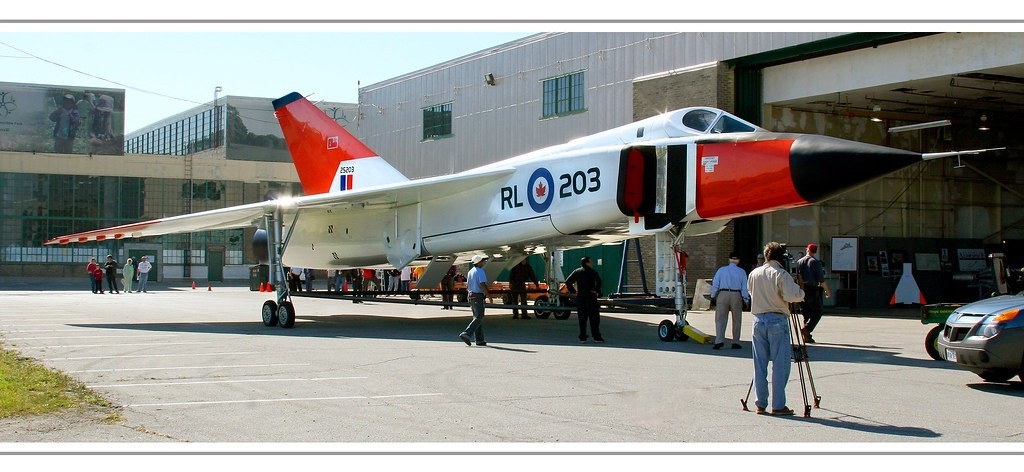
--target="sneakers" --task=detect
[773,406,794,415]
[756,406,766,413]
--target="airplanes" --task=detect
[40,91,1006,343]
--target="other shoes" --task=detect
[521,315,531,320]
[577,336,587,343]
[460,334,471,346]
[512,315,519,319]
[594,337,606,343]
[731,343,742,349]
[713,343,724,349]
[801,328,815,343]
[476,342,486,345]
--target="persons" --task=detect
[279,256,438,306]
[746,241,806,416]
[48,92,82,153]
[93,264,104,294]
[441,265,458,311]
[509,255,540,320]
[457,255,493,346]
[752,254,764,271]
[710,252,750,350]
[136,256,152,293]
[795,244,831,344]
[123,258,134,293]
[86,258,105,293]
[797,251,805,259]
[76,89,95,130]
[565,256,606,344]
[87,94,115,146]
[104,254,119,294]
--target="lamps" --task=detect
[978,114,990,131]
[888,118,952,133]
[870,100,883,123]
[484,73,496,86]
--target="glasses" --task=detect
[757,257,764,259]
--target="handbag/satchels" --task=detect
[121,279,126,285]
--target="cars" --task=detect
[937,288,1024,383]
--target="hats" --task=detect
[472,255,489,264]
[729,252,740,260]
[806,244,817,254]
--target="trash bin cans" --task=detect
[248,264,270,291]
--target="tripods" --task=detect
[741,291,822,418]
